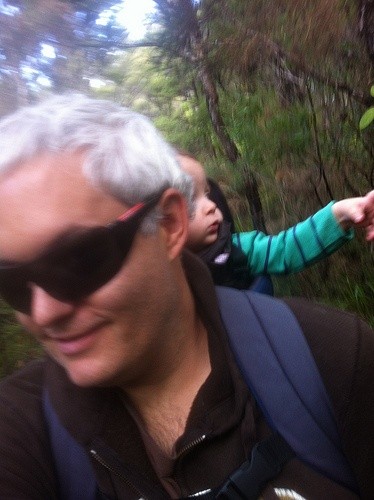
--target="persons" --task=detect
[176,150,374,294]
[2,90,373,499]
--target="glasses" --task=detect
[1,181,173,315]
